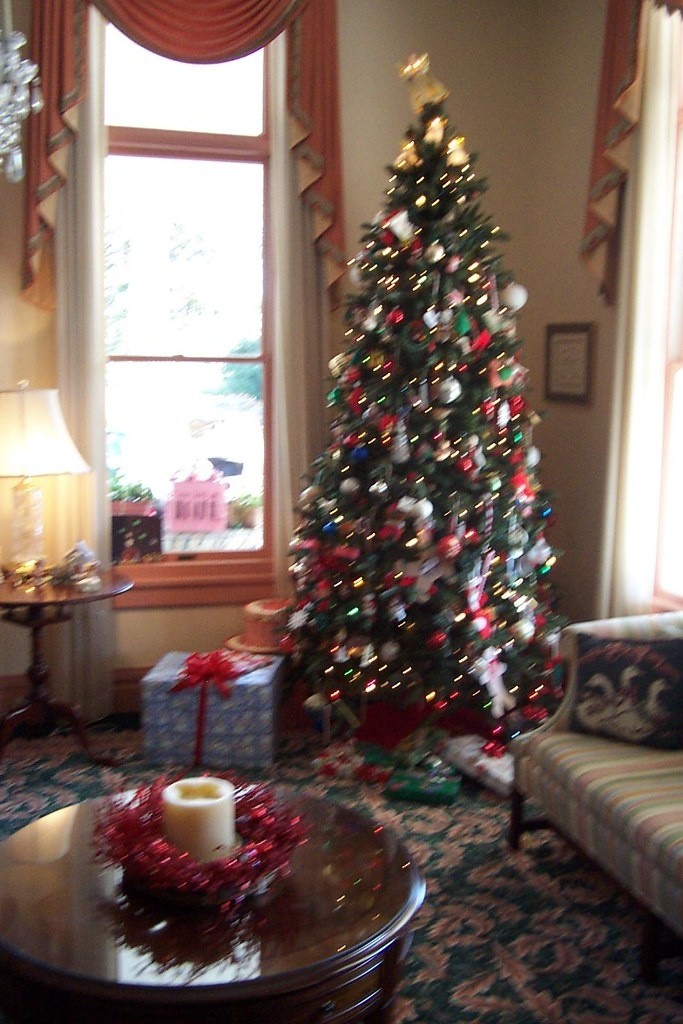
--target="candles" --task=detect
[161,777,244,859]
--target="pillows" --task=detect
[552,633,683,750]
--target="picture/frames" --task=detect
[544,322,595,407]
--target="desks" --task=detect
[0,573,135,759]
[0,789,427,1024]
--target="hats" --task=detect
[227,597,294,653]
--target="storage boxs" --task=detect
[112,502,162,559]
[139,652,286,770]
[302,693,515,803]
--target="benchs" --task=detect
[511,608,683,939]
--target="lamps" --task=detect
[0,387,92,575]
